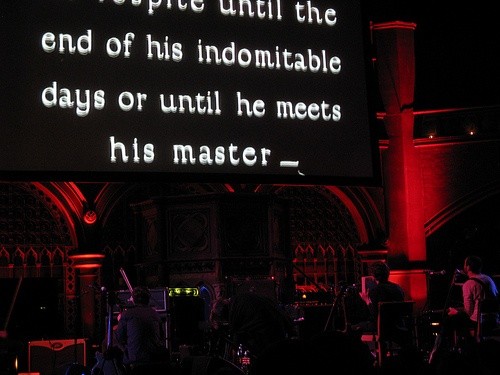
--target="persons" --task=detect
[447,256,500,336]
[115,286,171,365]
[331,280,353,337]
[350,261,405,338]
[175,270,288,370]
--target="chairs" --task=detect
[360,301,417,367]
[470,297,500,365]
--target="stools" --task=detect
[123,352,171,375]
